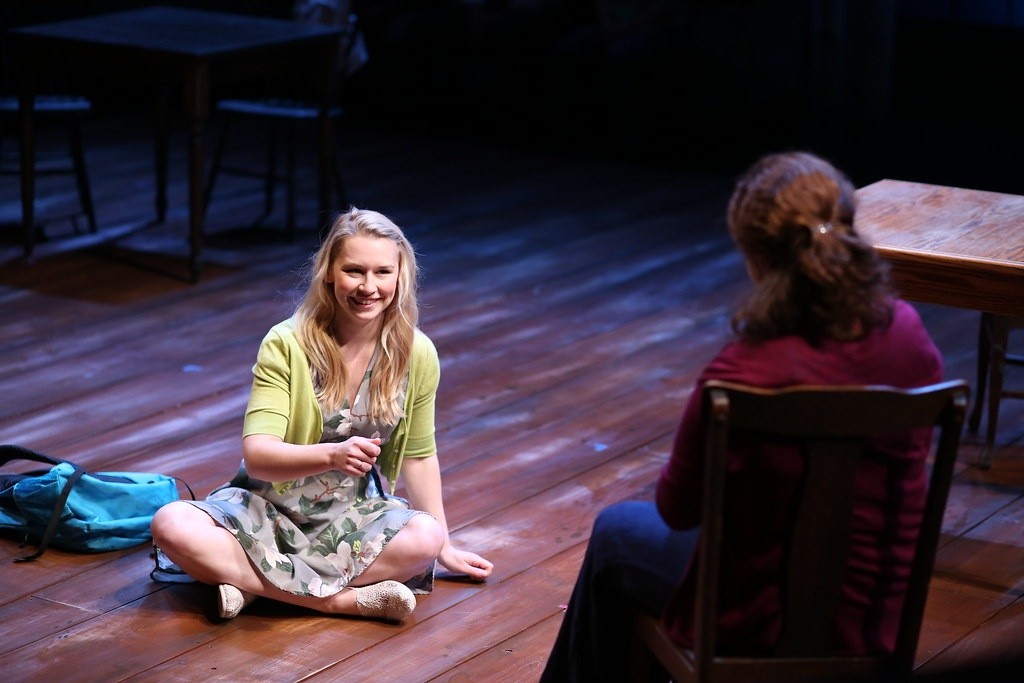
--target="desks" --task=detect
[854,178,1023,313]
[15,8,349,284]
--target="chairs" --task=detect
[0,97,97,253]
[201,2,358,240]
[635,377,972,683]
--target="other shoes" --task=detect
[218,584,256,619]
[346,579,417,621]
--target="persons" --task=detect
[151,211,493,623]
[537,152,943,683]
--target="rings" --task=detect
[359,463,363,469]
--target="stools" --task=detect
[967,311,1023,469]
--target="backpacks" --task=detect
[0,445,197,565]
